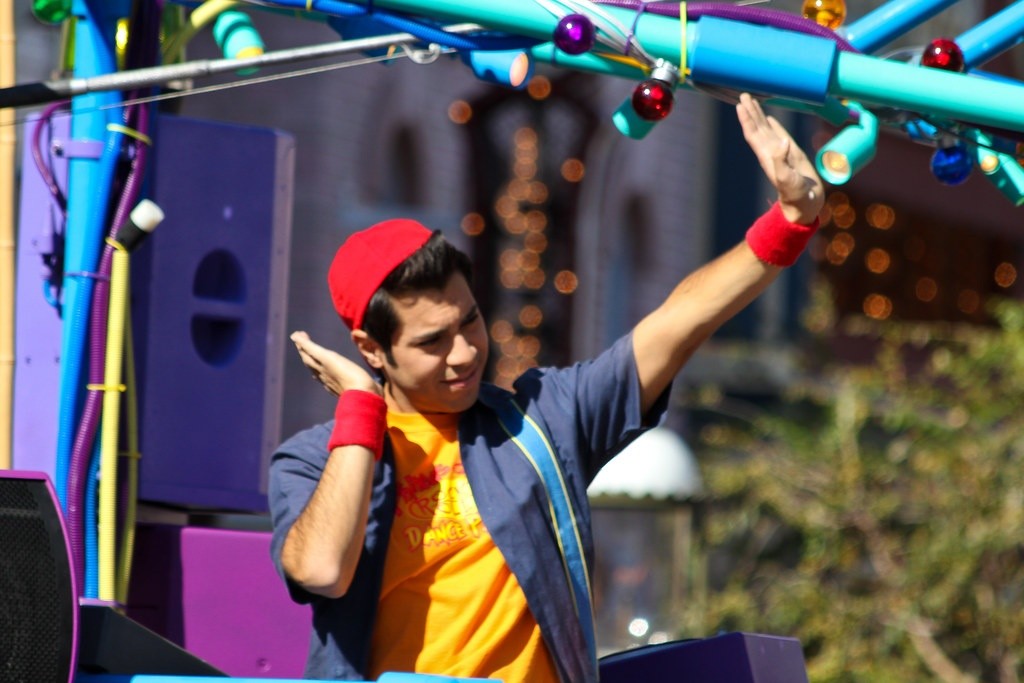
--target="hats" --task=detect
[327,218,432,330]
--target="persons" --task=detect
[268,91,825,683]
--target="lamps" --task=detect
[209,0,1024,208]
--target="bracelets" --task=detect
[746,204,819,270]
[327,390,387,460]
